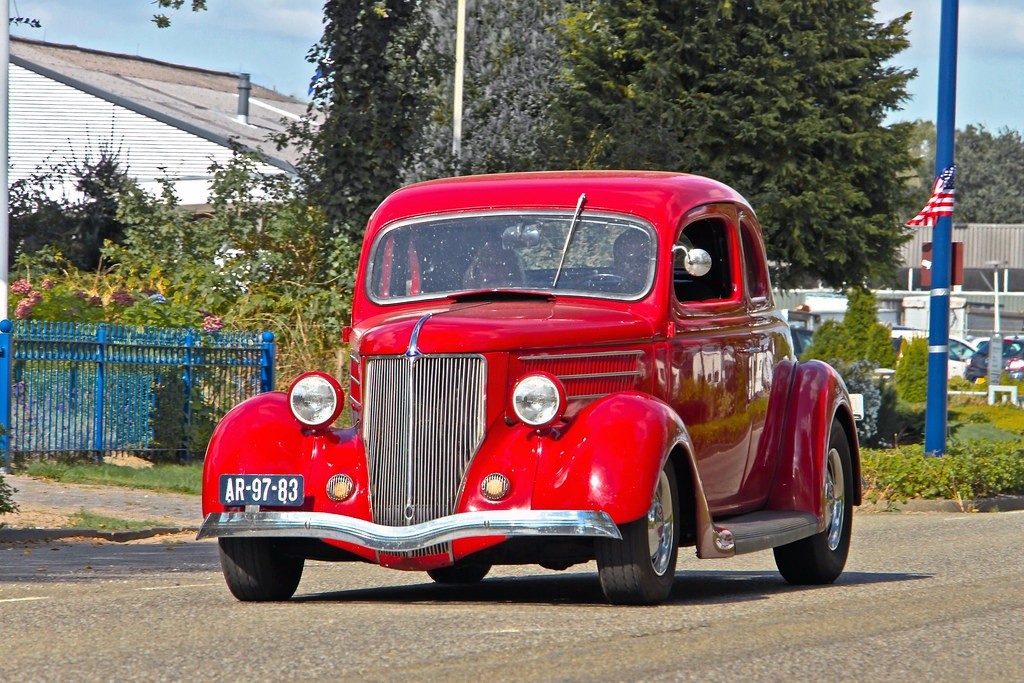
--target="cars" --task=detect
[780,304,1024,383]
[194,169,863,605]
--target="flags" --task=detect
[905,166,955,228]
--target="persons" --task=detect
[462,237,522,290]
[613,230,656,296]
